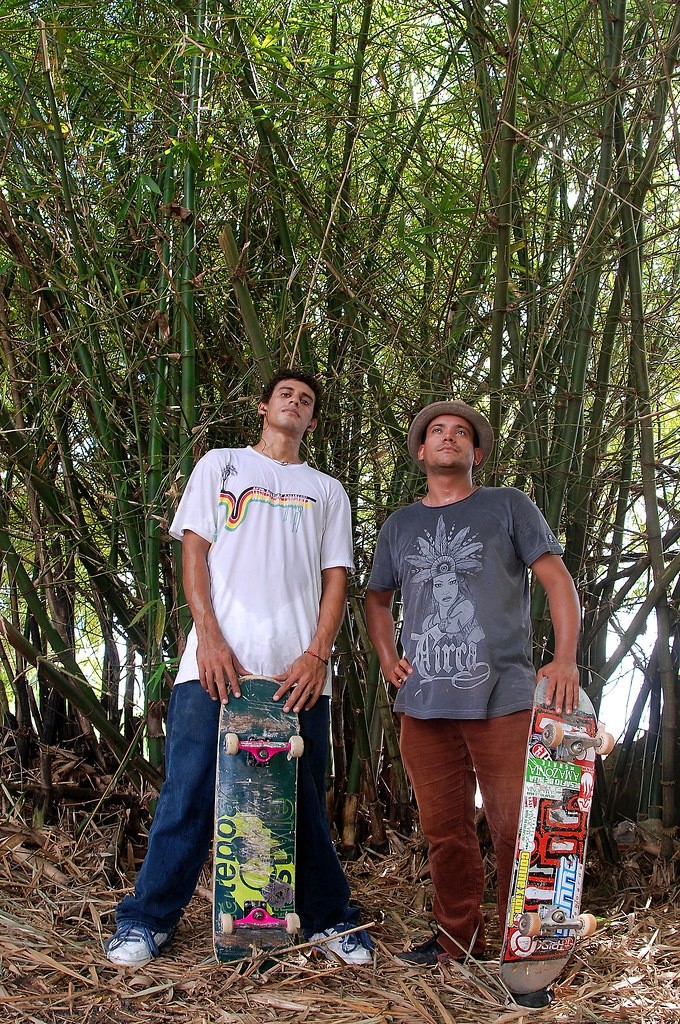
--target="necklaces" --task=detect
[426,486,476,506]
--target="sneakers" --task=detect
[505,987,555,1015]
[308,922,376,964]
[106,925,179,966]
[394,919,484,967]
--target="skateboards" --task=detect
[213,675,304,973]
[497,677,617,995]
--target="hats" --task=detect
[407,399,494,476]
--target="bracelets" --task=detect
[304,650,330,665]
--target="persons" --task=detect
[103,370,374,970]
[361,398,581,1013]
